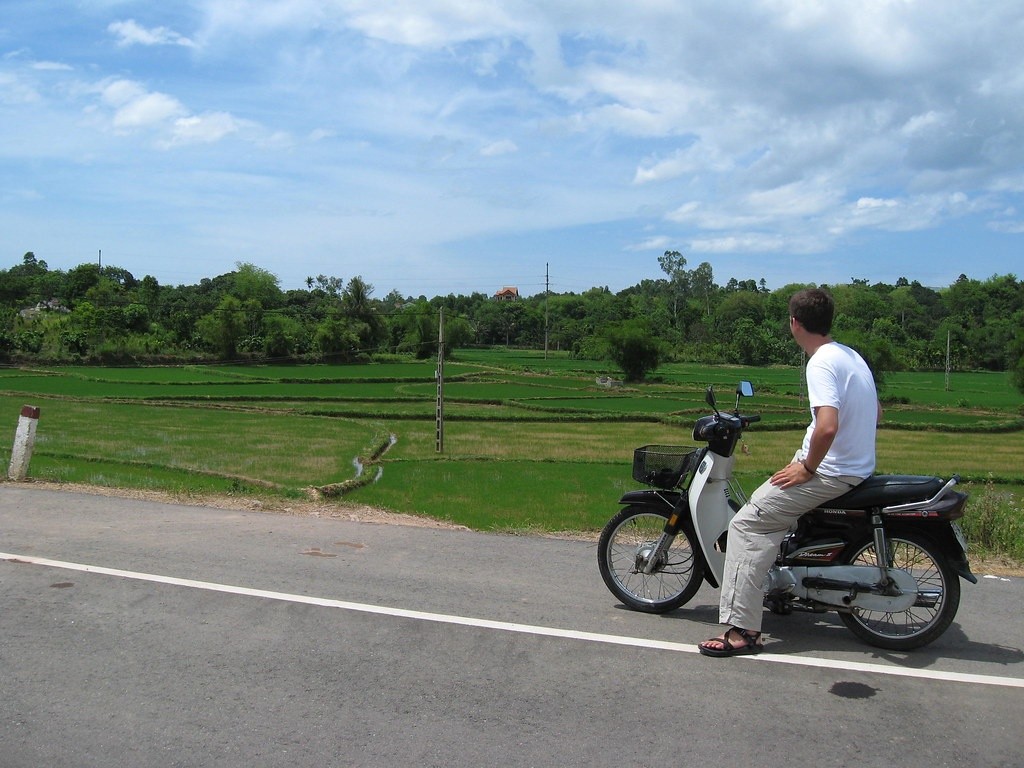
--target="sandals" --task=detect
[763,598,793,614]
[698,626,763,657]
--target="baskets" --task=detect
[632,445,698,490]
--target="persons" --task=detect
[698,289,882,657]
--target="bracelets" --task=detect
[804,464,814,474]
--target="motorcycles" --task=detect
[597,379,979,653]
